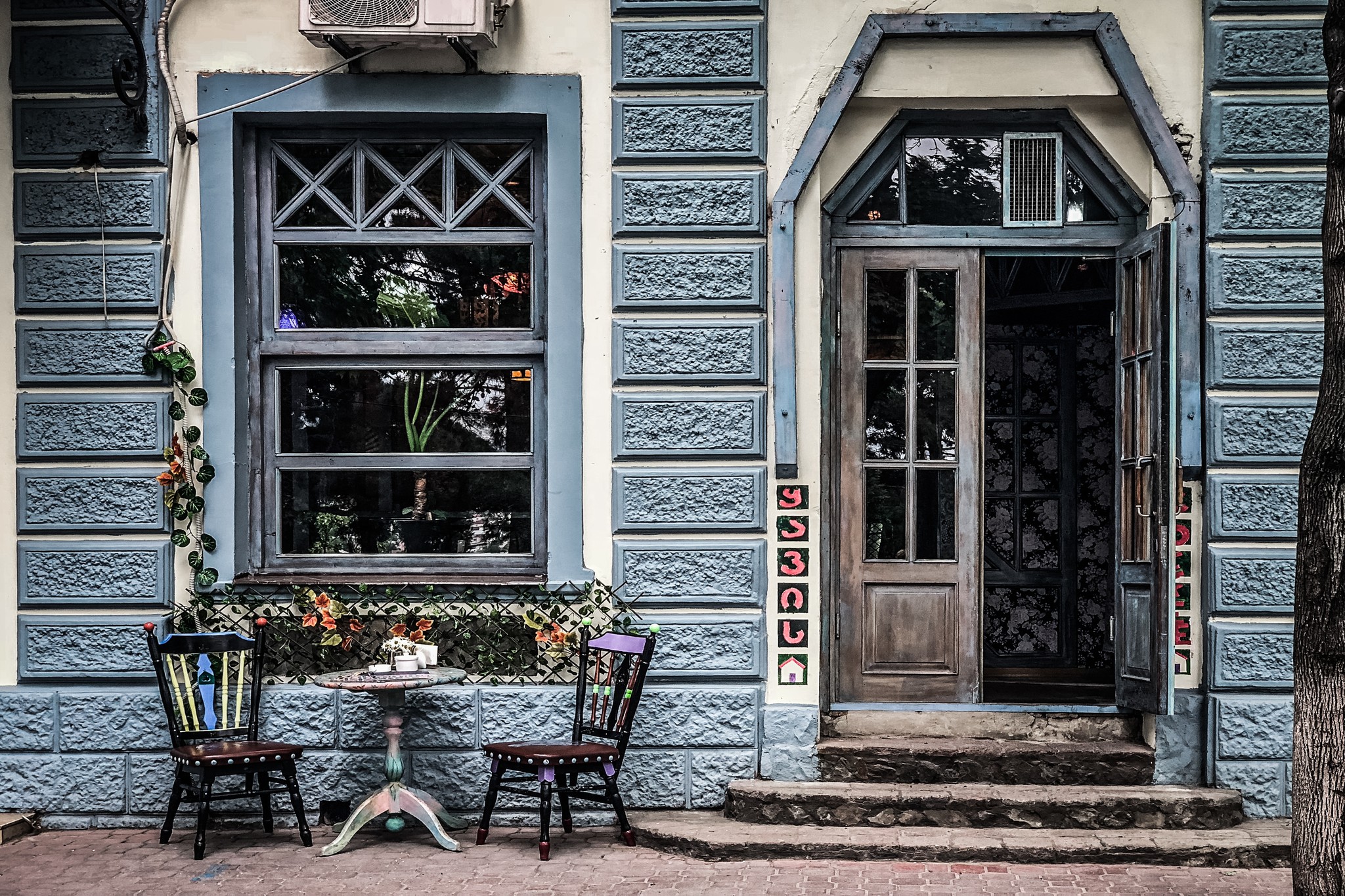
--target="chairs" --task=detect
[476,617,660,860]
[145,618,313,860]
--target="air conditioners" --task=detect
[298,0,516,50]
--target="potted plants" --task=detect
[371,371,468,554]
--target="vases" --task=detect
[390,650,403,669]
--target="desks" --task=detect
[315,666,468,854]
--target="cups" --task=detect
[394,652,419,672]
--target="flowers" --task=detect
[381,635,415,654]
